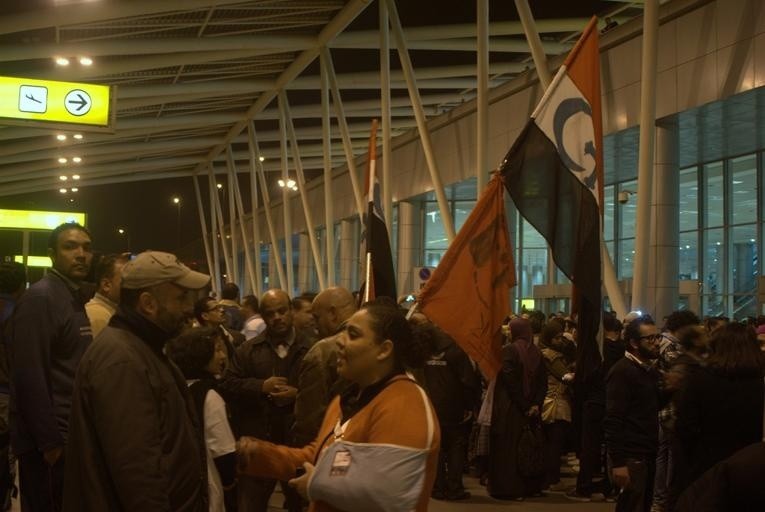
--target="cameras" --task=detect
[403,294,415,302]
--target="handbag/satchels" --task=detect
[516,412,544,475]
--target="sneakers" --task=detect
[566,488,591,502]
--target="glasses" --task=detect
[640,335,663,344]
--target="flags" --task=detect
[357,118,400,309]
[497,14,606,348]
[415,183,521,384]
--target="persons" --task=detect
[3,224,439,512]
[414,309,765,511]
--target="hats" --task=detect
[121,250,211,290]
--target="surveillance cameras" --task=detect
[618,192,629,204]
